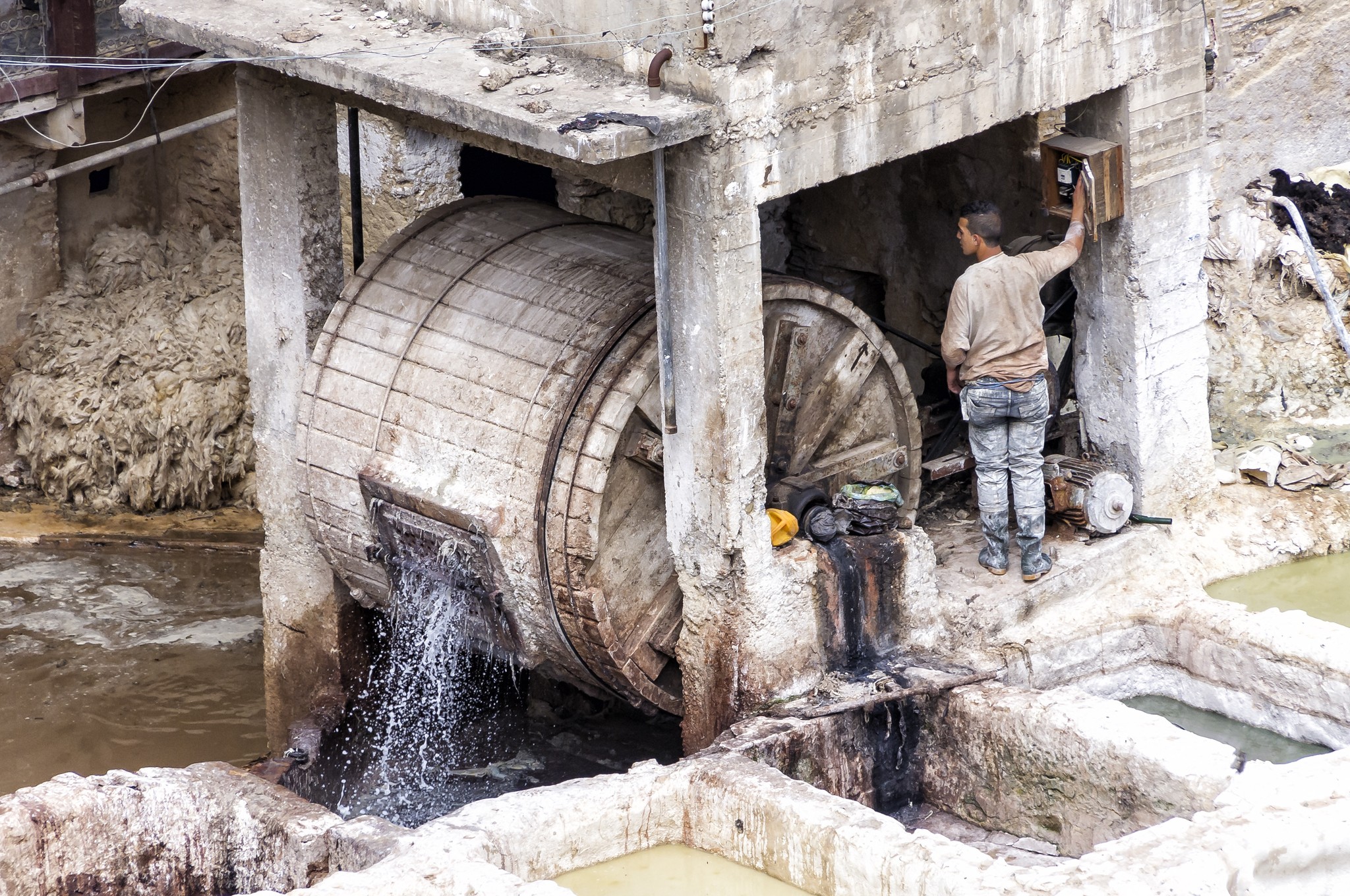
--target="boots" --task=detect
[1015,507,1053,580]
[978,508,1009,575]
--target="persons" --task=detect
[939,172,1089,583]
